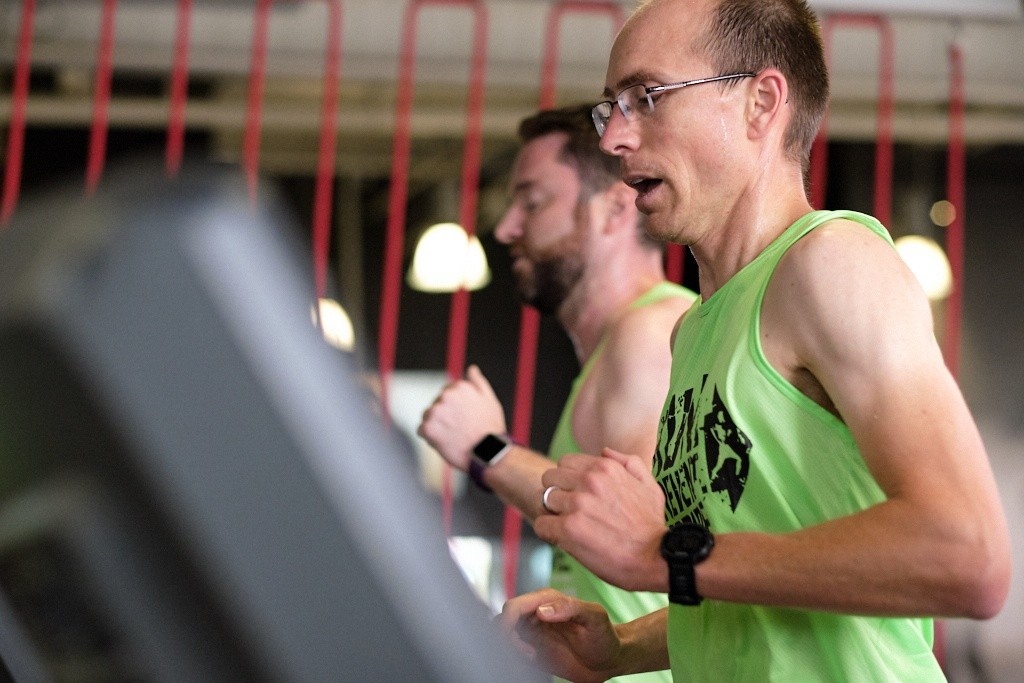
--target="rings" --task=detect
[544,486,557,508]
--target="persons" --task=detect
[416,104,703,683]
[493,0,1012,683]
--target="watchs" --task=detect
[468,433,512,494]
[660,522,714,606]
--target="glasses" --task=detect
[591,72,788,139]
